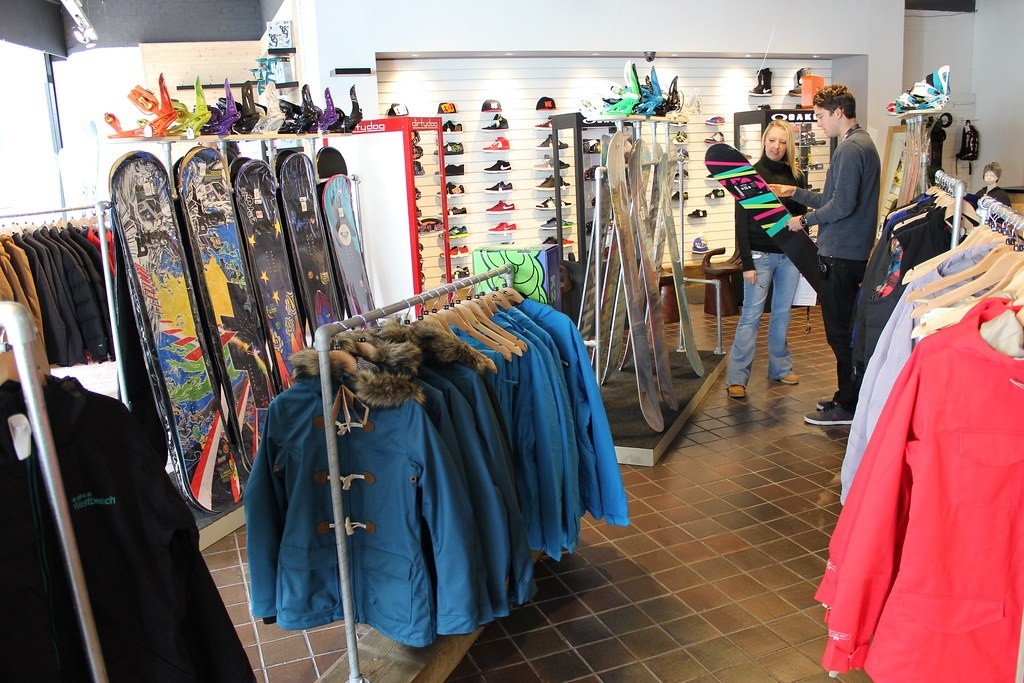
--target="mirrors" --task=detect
[876,125,907,241]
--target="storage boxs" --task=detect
[473,245,562,312]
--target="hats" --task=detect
[481,99,503,113]
[438,102,457,114]
[385,101,410,118]
[983,161,1002,180]
[536,96,557,111]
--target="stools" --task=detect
[659,268,735,323]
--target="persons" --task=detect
[767,84,881,425]
[975,162,1011,208]
[727,119,808,397]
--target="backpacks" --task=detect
[955,120,979,175]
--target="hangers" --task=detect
[320,272,570,387]
[0,330,124,416]
[887,172,1024,360]
[0,207,109,254]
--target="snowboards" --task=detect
[92,144,383,514]
[572,128,707,435]
[705,141,821,295]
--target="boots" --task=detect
[748,67,773,96]
[789,67,811,97]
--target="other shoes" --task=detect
[779,373,800,385]
[727,383,745,398]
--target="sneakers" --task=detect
[483,180,513,194]
[487,221,516,233]
[535,114,731,258]
[438,226,469,239]
[482,160,512,174]
[481,114,509,133]
[481,136,511,152]
[485,200,516,214]
[441,265,470,278]
[443,120,462,131]
[436,182,465,197]
[804,399,854,426]
[434,163,464,176]
[433,141,464,156]
[438,206,467,219]
[816,393,840,410]
[439,245,469,259]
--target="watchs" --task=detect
[799,215,807,228]
